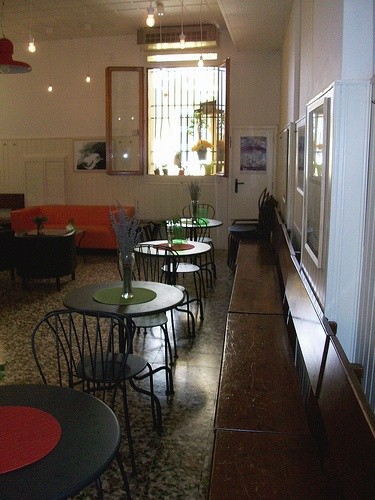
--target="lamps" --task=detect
[0,0,32,74]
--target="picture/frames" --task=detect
[73,139,108,172]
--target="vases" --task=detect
[191,200,198,223]
[119,251,135,299]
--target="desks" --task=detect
[167,216,223,243]
[133,238,212,312]
[63,281,186,405]
[0,385,132,500]
[29,228,87,262]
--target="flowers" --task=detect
[108,202,142,294]
[188,177,202,218]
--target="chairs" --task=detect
[0,204,216,500]
[227,188,279,270]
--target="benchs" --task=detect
[208,207,375,500]
[11,205,133,248]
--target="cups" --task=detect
[154,164,214,176]
